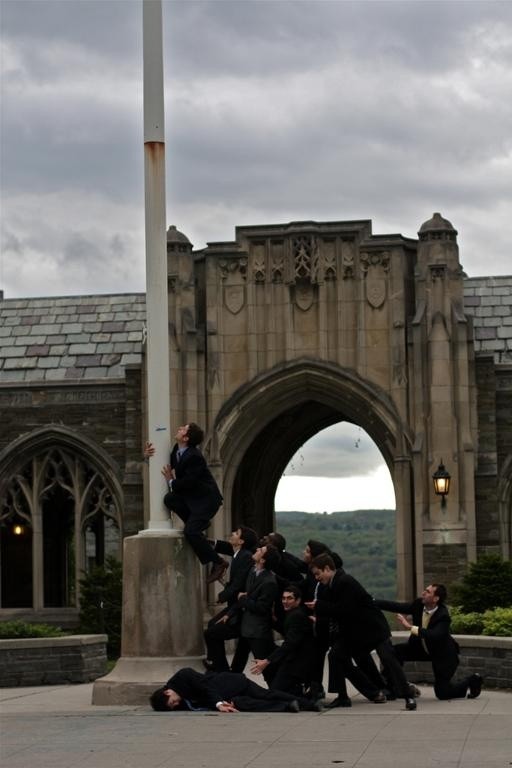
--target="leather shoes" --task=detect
[206,558,229,583]
[467,673,481,698]
[324,697,351,708]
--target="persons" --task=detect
[144,421,228,585]
[209,532,289,673]
[320,552,421,702]
[249,585,328,701]
[282,537,331,596]
[148,666,324,716]
[303,552,417,712]
[379,581,484,701]
[202,525,278,686]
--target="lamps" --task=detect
[431,457,452,509]
